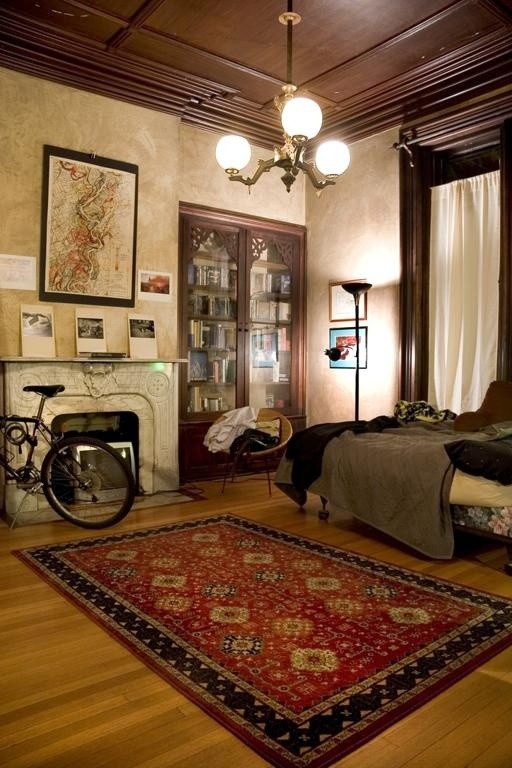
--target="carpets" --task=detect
[8,510,512,768]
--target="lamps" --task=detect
[214,0,351,197]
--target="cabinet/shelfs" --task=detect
[180,204,306,483]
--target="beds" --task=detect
[272,409,512,577]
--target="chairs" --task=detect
[203,404,294,496]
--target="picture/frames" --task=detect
[37,143,139,310]
[328,278,369,323]
[328,325,369,370]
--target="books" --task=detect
[188,320,236,348]
[250,297,292,321]
[188,265,237,288]
[250,273,292,294]
[251,324,291,352]
[188,351,235,384]
[279,373,290,382]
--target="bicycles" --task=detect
[0,384,136,533]
